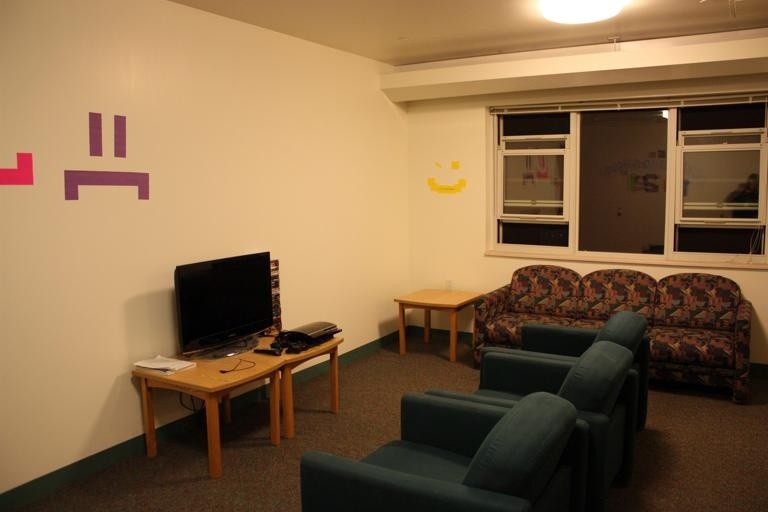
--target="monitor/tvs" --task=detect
[173,251,274,359]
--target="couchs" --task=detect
[301,391,591,512]
[426,310,651,511]
[473,264,755,408]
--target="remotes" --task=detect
[254,349,281,356]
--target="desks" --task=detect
[133,334,344,481]
[392,285,482,365]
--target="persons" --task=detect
[724,172,759,218]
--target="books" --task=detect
[133,354,198,376]
